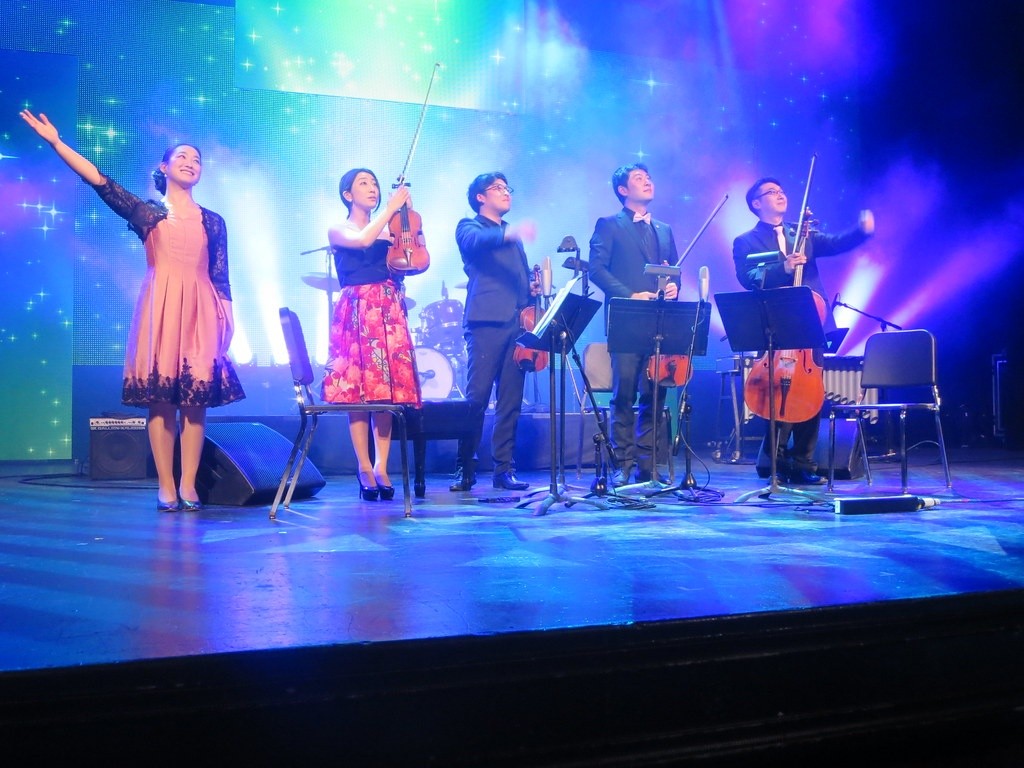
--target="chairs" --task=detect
[828,329,952,494]
[269,308,413,519]
[580,343,675,483]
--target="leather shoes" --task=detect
[791,467,828,484]
[179,492,201,511]
[767,472,787,484]
[157,498,179,512]
[449,467,477,490]
[493,472,529,490]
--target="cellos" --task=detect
[743,206,827,459]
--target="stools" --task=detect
[366,399,468,497]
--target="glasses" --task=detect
[751,188,787,200]
[479,183,514,194]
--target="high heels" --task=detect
[357,468,379,500]
[378,484,395,497]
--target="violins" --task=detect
[385,182,430,276]
[511,263,549,372]
[647,259,694,388]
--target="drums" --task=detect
[423,298,465,340]
[413,346,464,402]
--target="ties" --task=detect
[773,226,785,257]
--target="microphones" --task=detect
[418,370,435,379]
[830,294,839,312]
[698,266,710,302]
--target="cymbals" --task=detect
[303,275,343,292]
[454,284,468,289]
[403,298,418,309]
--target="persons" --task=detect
[448,172,539,492]
[587,164,681,487]
[320,169,423,501]
[733,177,874,485]
[20,109,247,512]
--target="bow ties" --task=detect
[633,211,651,225]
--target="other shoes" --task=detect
[611,466,629,487]
[634,470,672,485]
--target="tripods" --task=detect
[513,272,829,515]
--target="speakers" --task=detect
[88,416,158,480]
[173,423,326,506]
[756,419,865,480]
